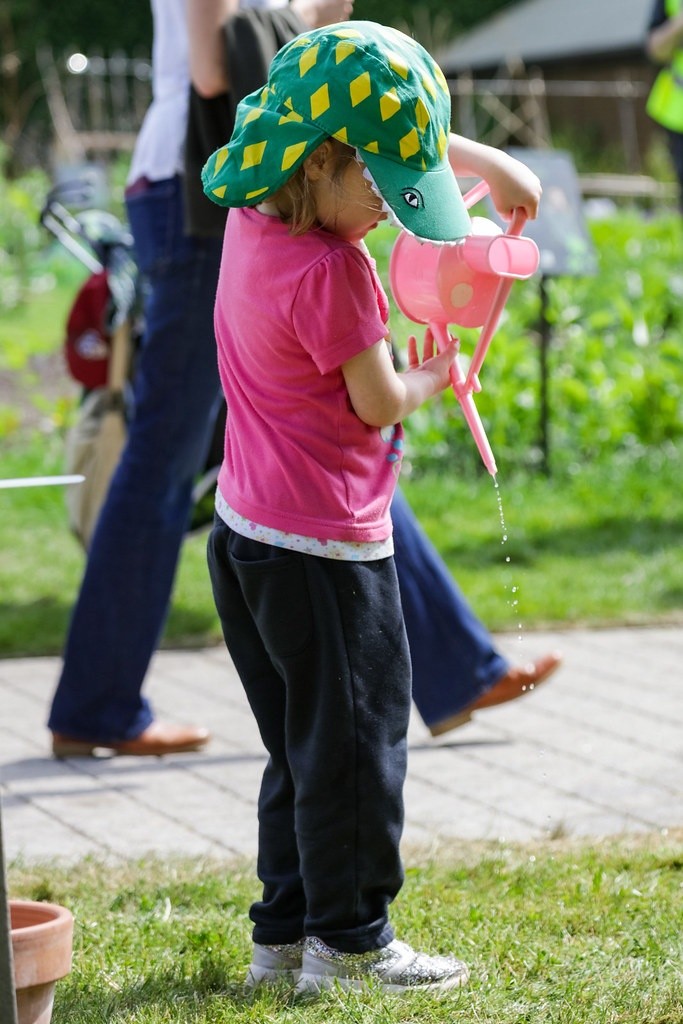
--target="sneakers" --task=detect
[246,937,306,988]
[296,937,469,996]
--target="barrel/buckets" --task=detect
[7,901,74,1024]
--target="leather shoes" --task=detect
[430,657,559,735]
[52,725,209,758]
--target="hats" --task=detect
[200,21,472,247]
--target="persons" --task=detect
[644,0,683,218]
[45,0,560,764]
[200,19,543,997]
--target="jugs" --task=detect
[390,179,538,470]
